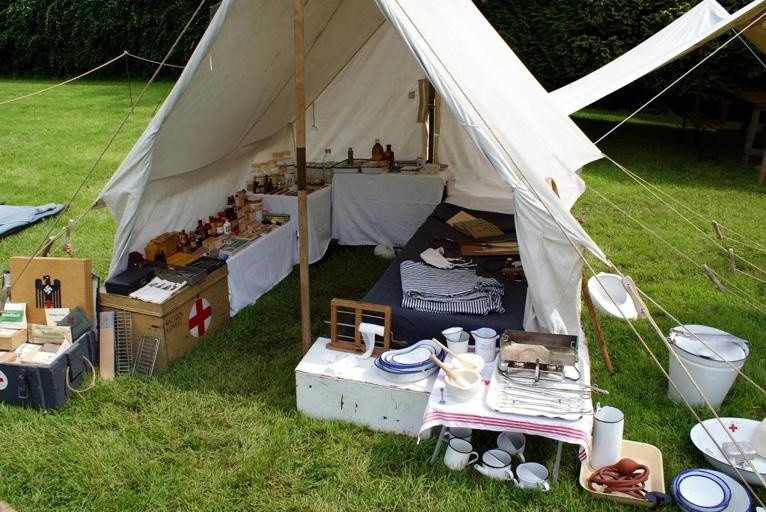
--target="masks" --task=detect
[420,247,478,269]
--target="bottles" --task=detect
[180,229,188,246]
[194,230,202,248]
[372,138,383,161]
[348,147,353,164]
[216,223,223,235]
[197,219,205,241]
[190,237,196,252]
[223,219,232,235]
[227,196,233,208]
[322,148,335,184]
[209,218,218,237]
[231,195,235,205]
[224,207,240,236]
[204,220,212,237]
[252,174,274,194]
[383,144,395,164]
[247,194,264,222]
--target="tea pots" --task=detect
[474,449,514,481]
[497,431,525,462]
[513,463,550,492]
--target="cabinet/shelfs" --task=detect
[225,219,293,316]
[333,168,448,245]
[246,186,332,262]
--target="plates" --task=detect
[392,347,432,365]
[385,344,436,365]
[185,256,226,275]
[379,354,442,372]
[157,264,207,286]
[360,160,391,174]
[398,165,422,175]
[331,158,369,173]
[374,352,443,383]
[579,436,665,508]
[380,339,442,367]
[486,352,586,421]
[219,240,250,256]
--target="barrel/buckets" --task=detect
[668,325,749,412]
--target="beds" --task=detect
[348,201,526,349]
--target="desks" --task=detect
[427,333,591,486]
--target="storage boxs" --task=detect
[98,262,229,369]
[0,324,94,409]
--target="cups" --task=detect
[590,406,624,470]
[470,327,500,362]
[447,331,475,353]
[442,427,473,443]
[444,437,479,470]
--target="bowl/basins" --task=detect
[673,474,729,512]
[444,369,481,403]
[670,469,752,512]
[691,417,765,485]
[451,352,485,372]
[676,470,732,508]
[423,163,440,173]
[678,474,726,507]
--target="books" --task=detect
[446,210,504,239]
